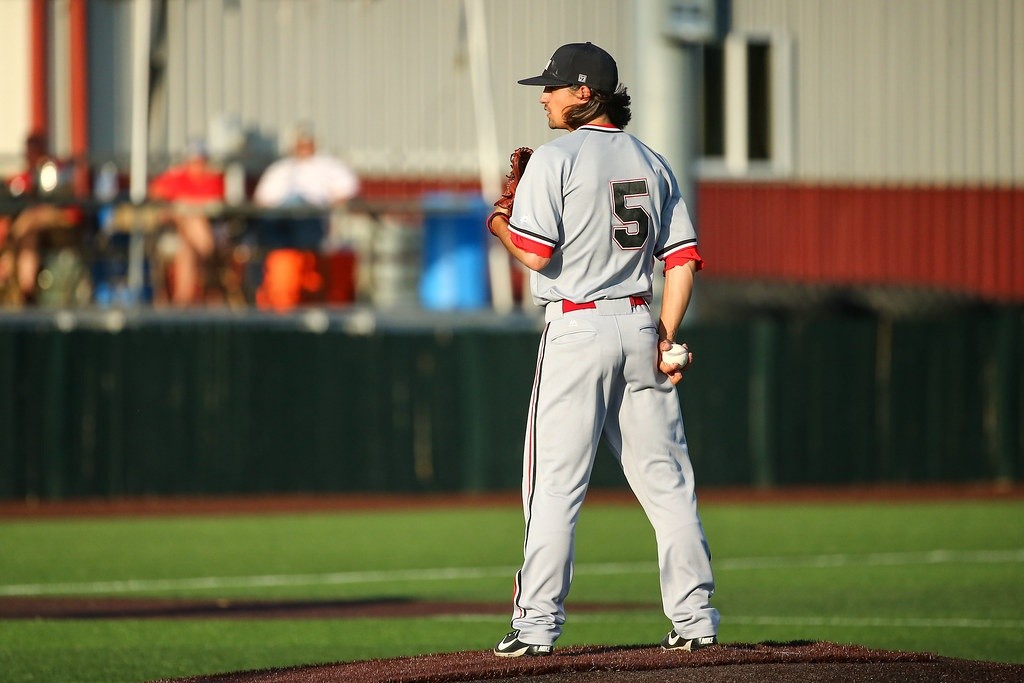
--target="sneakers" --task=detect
[493,629,553,658]
[656,626,718,652]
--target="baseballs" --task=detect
[661,344,689,369]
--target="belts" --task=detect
[562,296,645,313]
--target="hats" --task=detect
[517,42,618,93]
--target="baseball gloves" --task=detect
[497,147,534,217]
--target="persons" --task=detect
[492,41,720,657]
[0,120,359,313]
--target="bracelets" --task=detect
[486,211,509,237]
